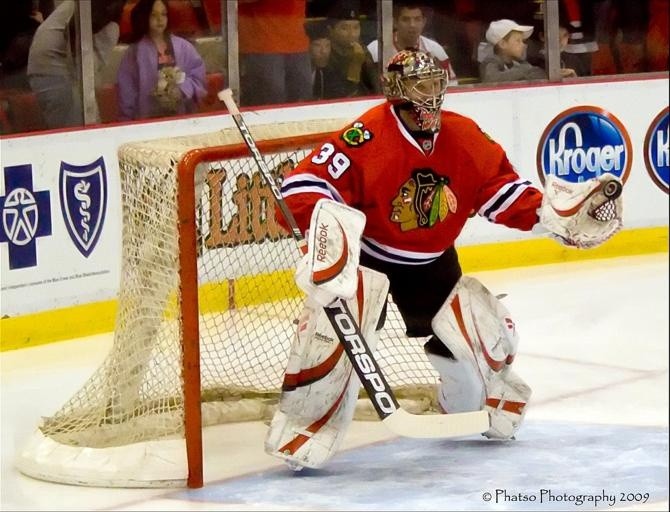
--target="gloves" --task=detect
[294,200,366,306]
[541,173,624,248]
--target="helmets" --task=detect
[384,49,448,105]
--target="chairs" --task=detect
[0,1,670,133]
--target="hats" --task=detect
[305,25,328,37]
[485,19,534,45]
[328,7,360,19]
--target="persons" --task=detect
[262,45,624,474]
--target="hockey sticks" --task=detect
[217,88,491,439]
